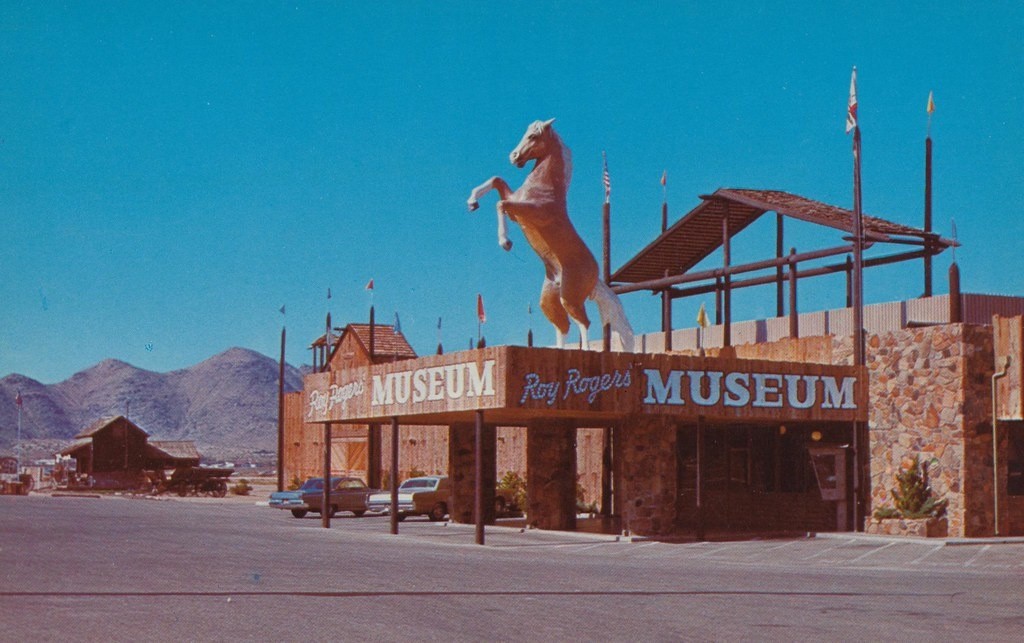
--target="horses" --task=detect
[467,116,635,352]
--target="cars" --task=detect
[270,475,381,518]
[365,474,515,523]
